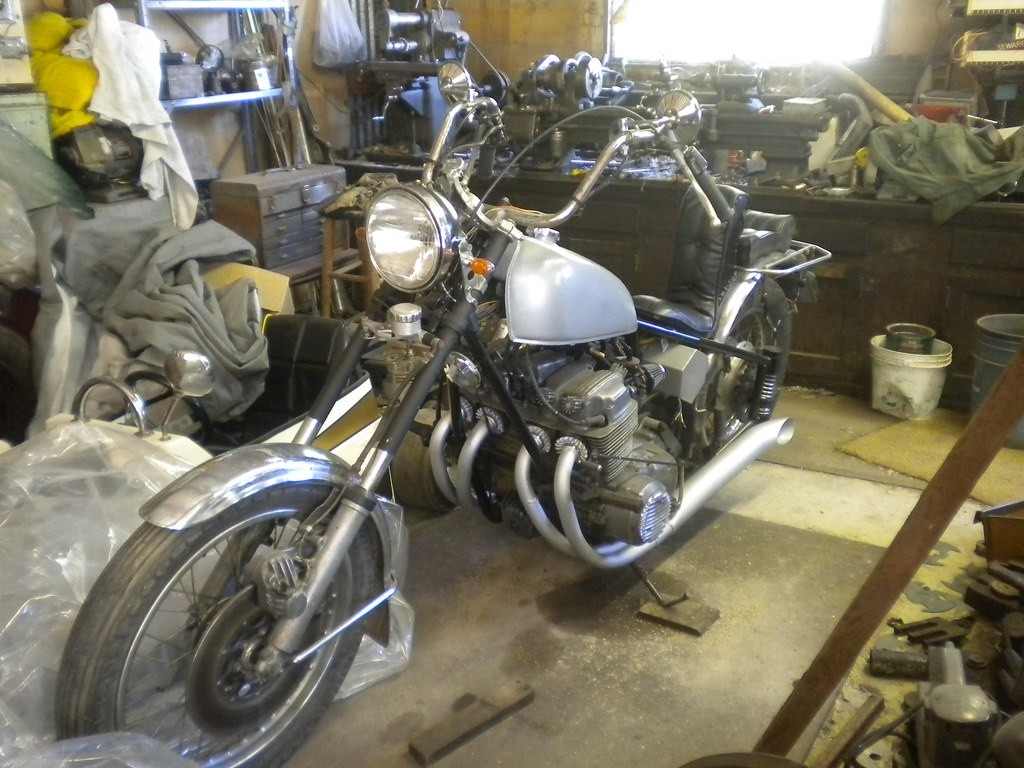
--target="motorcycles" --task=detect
[53,60,833,768]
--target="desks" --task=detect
[268,247,361,313]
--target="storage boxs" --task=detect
[0,91,61,212]
[210,161,350,271]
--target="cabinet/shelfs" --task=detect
[336,148,1024,411]
[136,0,305,166]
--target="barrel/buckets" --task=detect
[871,334,954,421]
[888,322,936,352]
[973,313,1024,441]
